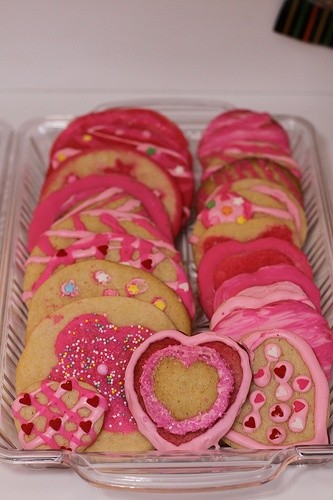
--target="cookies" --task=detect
[12,108,333,452]
